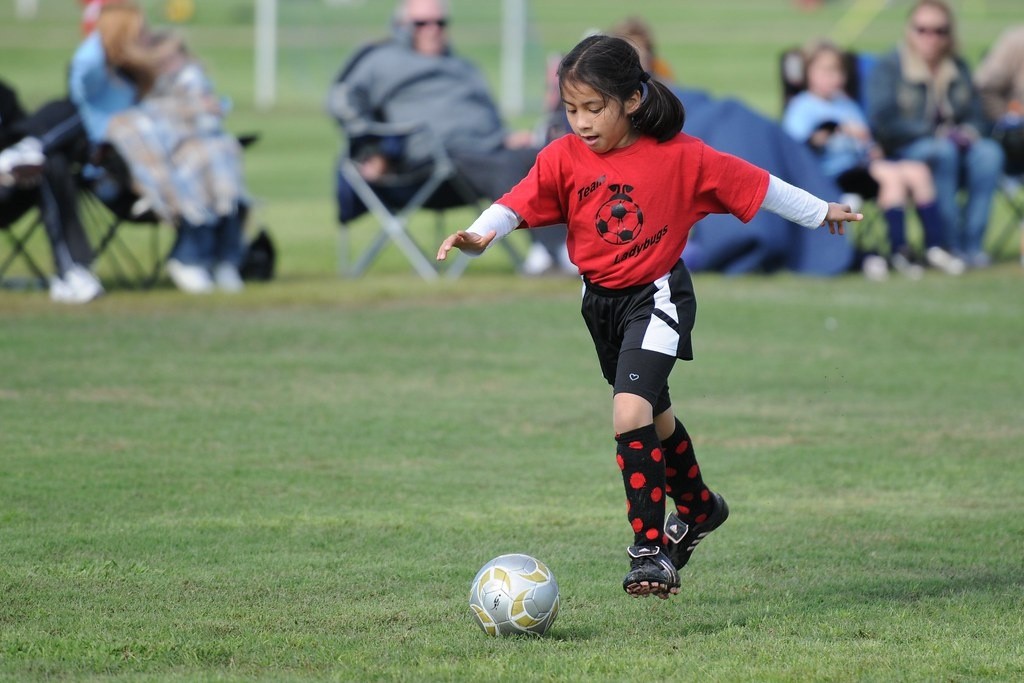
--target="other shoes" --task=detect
[167,258,214,293]
[862,252,885,283]
[925,246,967,276]
[214,263,247,294]
[51,267,107,305]
[522,242,551,276]
[891,251,921,279]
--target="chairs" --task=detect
[781,51,1024,269]
[0,80,260,290]
[336,117,528,282]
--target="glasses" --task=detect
[906,24,952,38]
[414,16,448,29]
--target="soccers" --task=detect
[467,553,561,641]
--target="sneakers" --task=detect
[622,544,681,600]
[663,488,731,571]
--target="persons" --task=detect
[69,0,254,291]
[437,33,864,598]
[778,41,967,278]
[608,17,674,82]
[866,0,1024,273]
[327,0,581,278]
[0,79,103,303]
